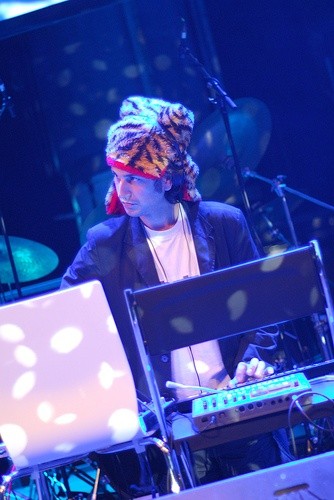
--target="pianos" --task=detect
[172,358,334,491]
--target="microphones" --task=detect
[180,17,187,60]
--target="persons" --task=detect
[59,96,282,487]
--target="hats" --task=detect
[105,95,202,216]
